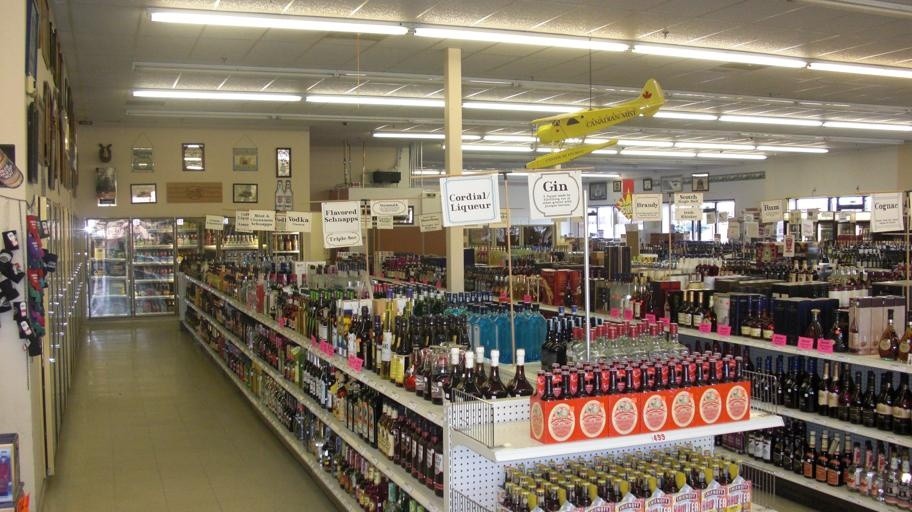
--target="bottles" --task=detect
[272,179,295,215]
[87,215,912,511]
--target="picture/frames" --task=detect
[612,180,621,191]
[96,167,117,206]
[130,184,157,203]
[589,181,607,201]
[642,179,652,191]
[26,0,80,190]
[691,172,709,192]
[232,183,259,203]
